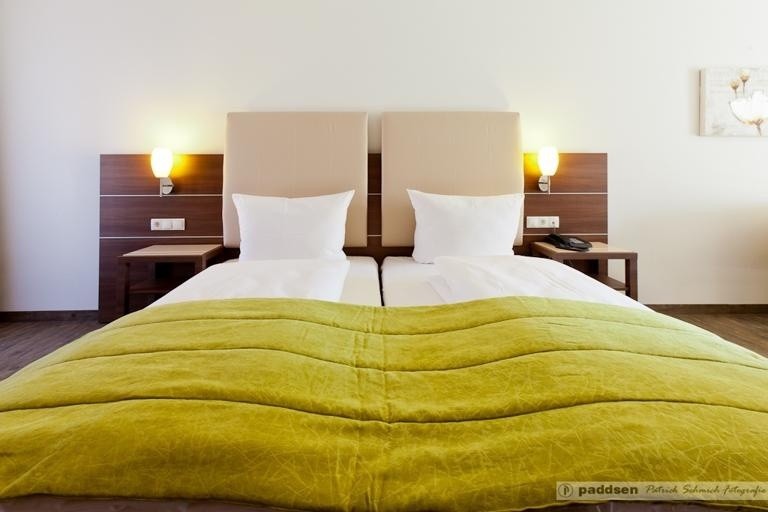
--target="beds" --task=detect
[0,111,767,510]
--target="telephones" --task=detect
[545,233,592,249]
